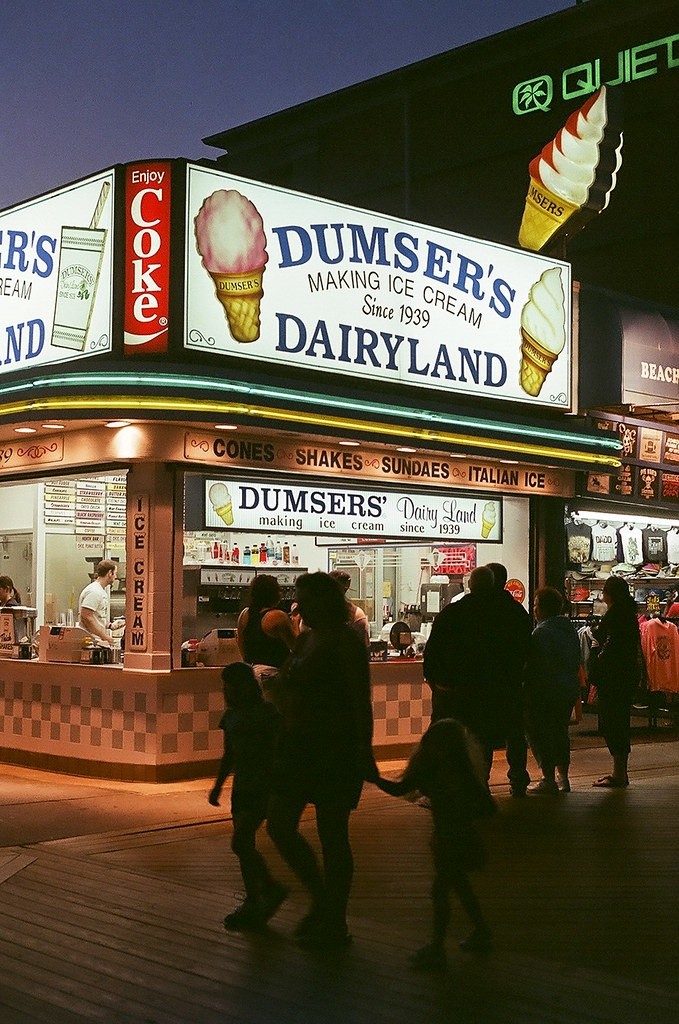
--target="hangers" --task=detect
[626,522,634,527]
[569,614,603,625]
[673,526,679,530]
[644,609,666,622]
[647,524,660,530]
[599,520,607,525]
[573,518,583,523]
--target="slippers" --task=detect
[593,774,629,786]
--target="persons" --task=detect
[209,569,497,964]
[423,563,581,794]
[585,576,639,787]
[79,560,117,646]
[0,576,21,607]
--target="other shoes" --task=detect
[527,778,558,793]
[508,786,526,798]
[458,932,495,954]
[225,882,288,931]
[417,942,447,963]
[556,778,570,792]
[296,915,351,943]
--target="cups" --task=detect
[50,225,107,352]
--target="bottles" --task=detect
[274,541,282,565]
[283,541,290,565]
[291,544,299,564]
[196,536,274,566]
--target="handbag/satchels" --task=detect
[587,644,612,680]
